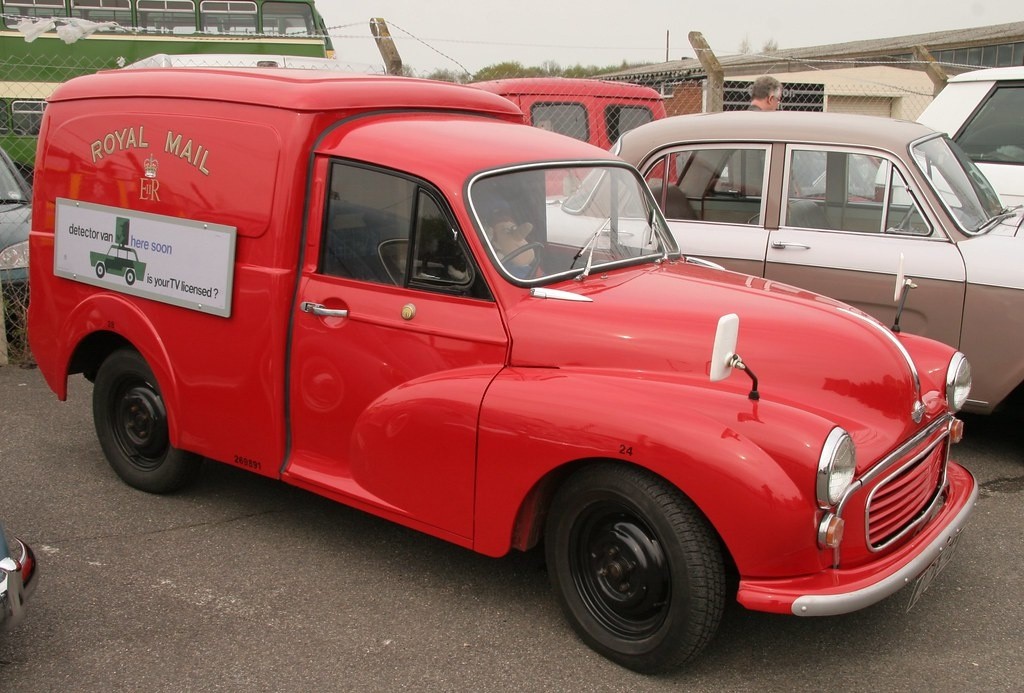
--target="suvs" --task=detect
[875,66,1024,208]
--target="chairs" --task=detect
[748,199,831,228]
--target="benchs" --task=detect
[650,185,699,220]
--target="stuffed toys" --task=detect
[483,210,546,280]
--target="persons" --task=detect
[727,77,782,196]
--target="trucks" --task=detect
[24,68,983,675]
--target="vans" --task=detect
[469,80,678,196]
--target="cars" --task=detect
[0,147,35,327]
[541,113,1024,453]
